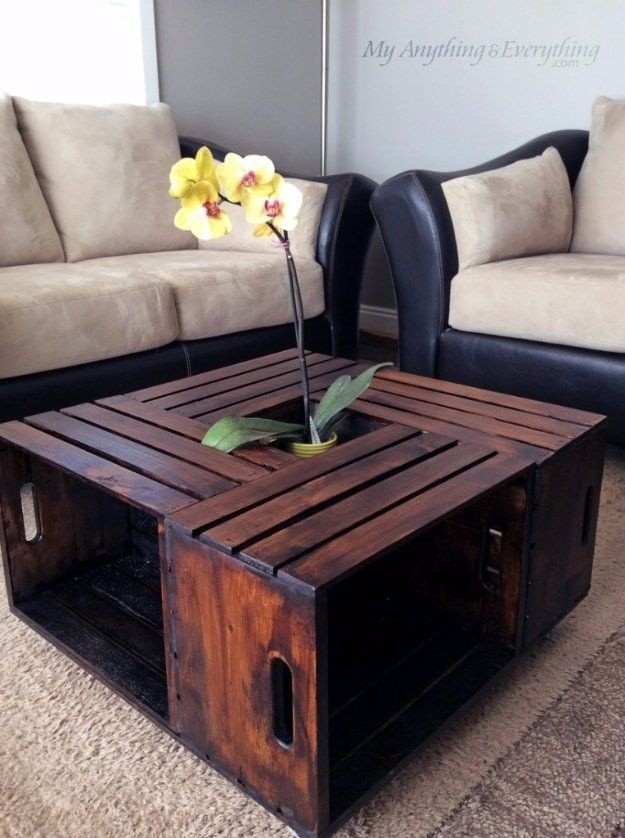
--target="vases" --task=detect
[268,432,337,459]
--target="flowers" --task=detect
[167,145,394,455]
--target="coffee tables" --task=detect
[0,346,606,838]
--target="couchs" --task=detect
[368,93,625,446]
[0,91,379,424]
